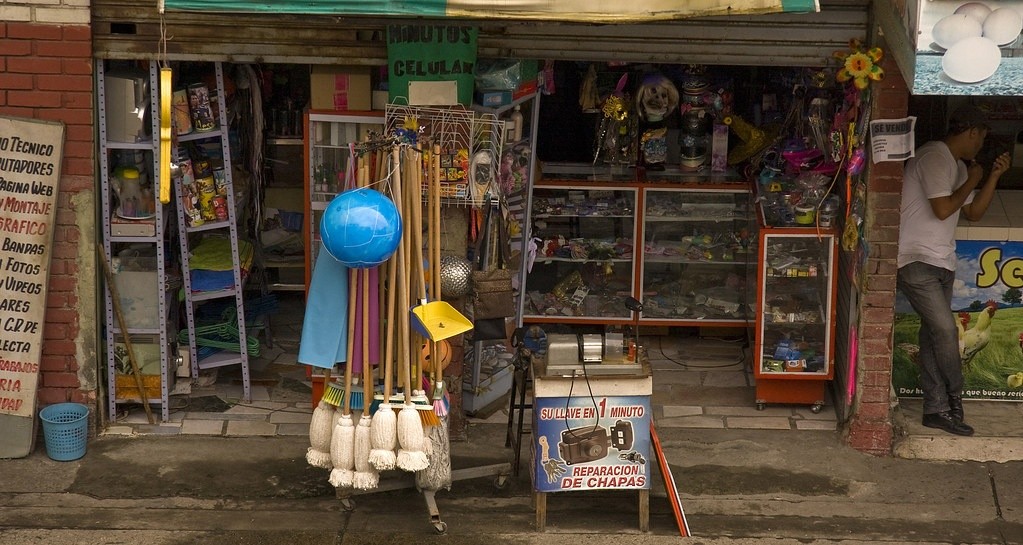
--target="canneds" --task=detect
[172,82,220,136]
[178,143,228,228]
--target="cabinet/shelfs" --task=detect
[520,176,839,414]
[95,57,385,424]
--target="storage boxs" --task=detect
[308,64,373,111]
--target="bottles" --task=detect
[142,188,155,214]
[119,169,144,216]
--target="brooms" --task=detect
[319,112,451,427]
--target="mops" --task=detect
[304,143,452,492]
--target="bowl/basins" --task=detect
[779,190,840,227]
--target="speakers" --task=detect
[104,66,151,143]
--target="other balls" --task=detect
[318,185,404,268]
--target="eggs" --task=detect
[933,3,1023,83]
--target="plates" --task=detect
[116,207,155,220]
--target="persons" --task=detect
[897,108,1011,437]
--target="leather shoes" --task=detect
[949,397,964,422]
[923,410,974,435]
